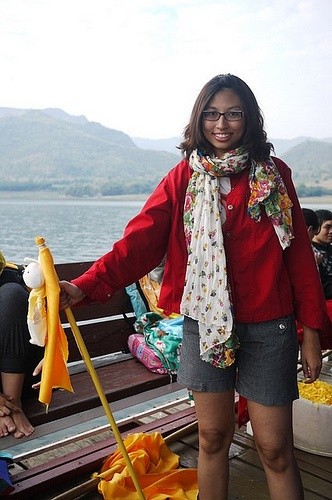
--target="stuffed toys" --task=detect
[21,262,47,347]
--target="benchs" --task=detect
[0,259,239,500]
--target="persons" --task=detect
[59,74,327,500]
[298,208,332,352]
[0,251,57,439]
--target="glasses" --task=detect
[202,109,246,122]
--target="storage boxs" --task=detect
[246,378,332,458]
